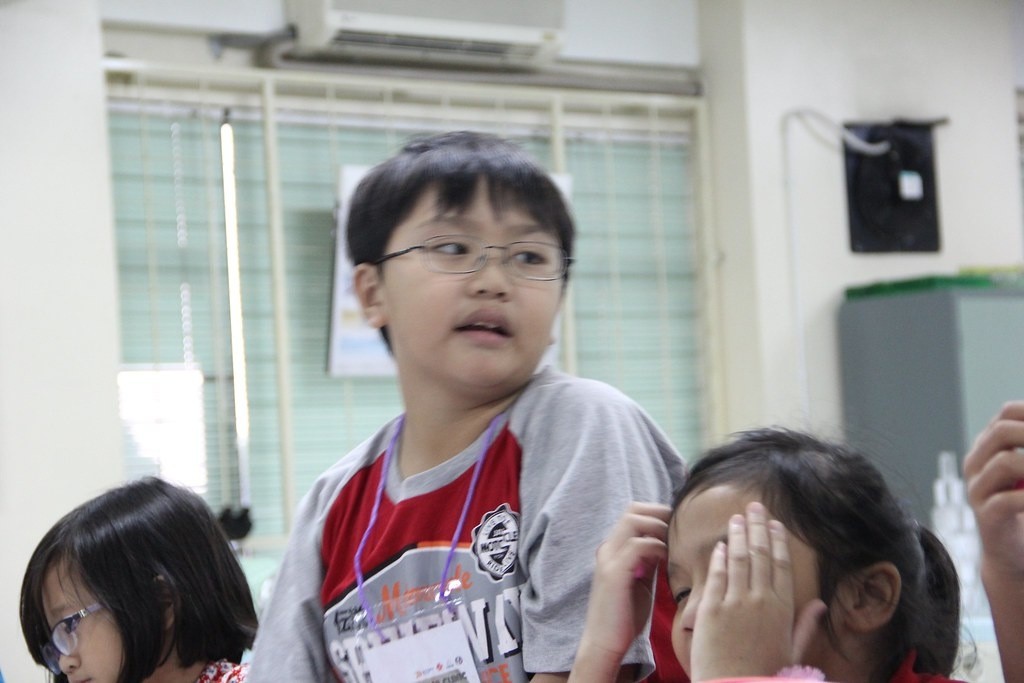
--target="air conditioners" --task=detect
[284,0,565,69]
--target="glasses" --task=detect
[377,234,572,280]
[41,602,108,674]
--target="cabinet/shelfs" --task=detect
[833,287,1024,529]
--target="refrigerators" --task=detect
[834,291,1024,531]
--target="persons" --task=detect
[241,132,689,683]
[567,426,970,683]
[19,478,259,682]
[965,397,1024,683]
